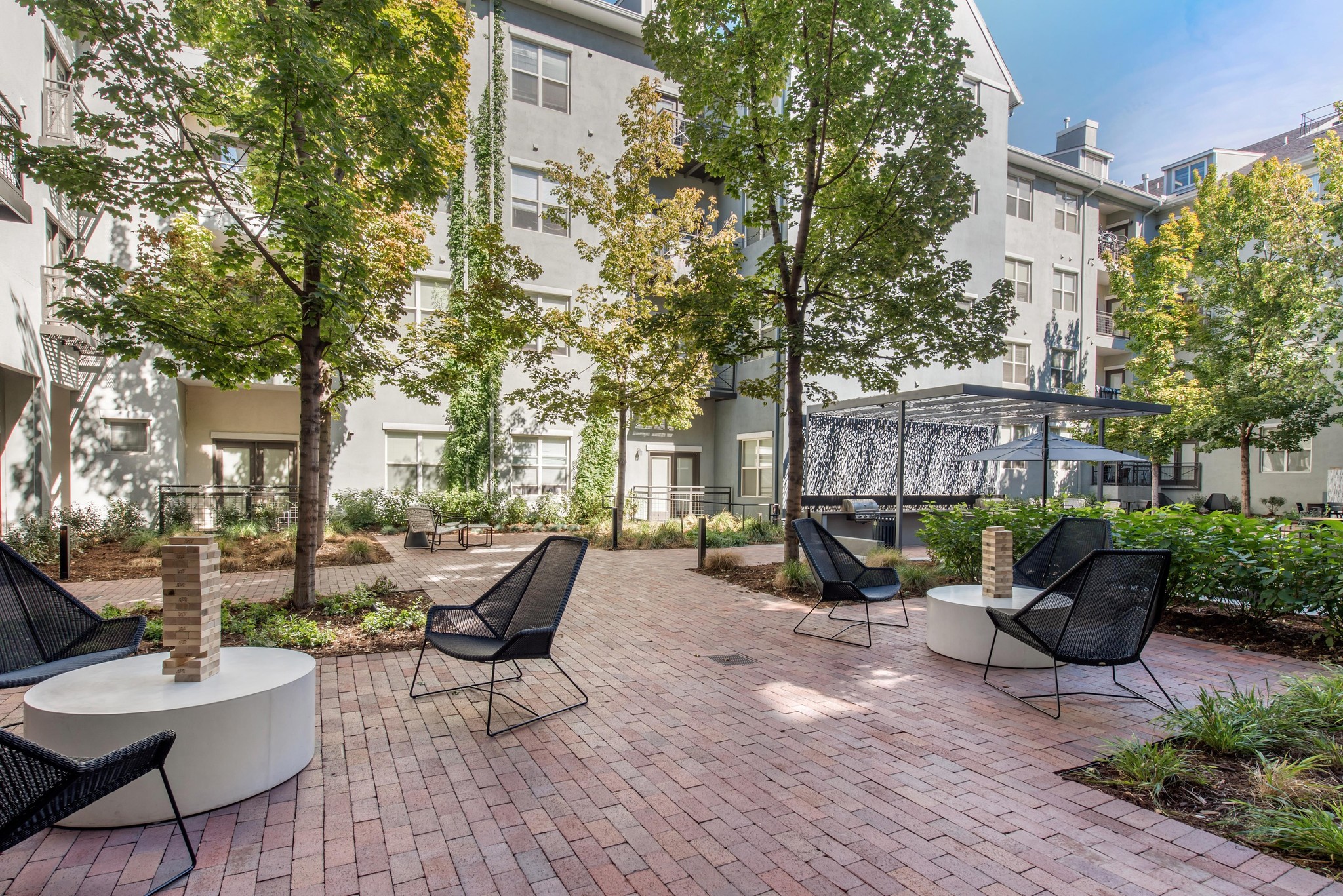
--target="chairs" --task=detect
[1010,517,1119,633]
[980,495,1343,534]
[0,724,199,896]
[402,503,495,552]
[407,534,593,738]
[983,546,1183,723]
[786,516,909,649]
[0,536,149,695]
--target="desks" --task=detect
[19,645,317,829]
[925,585,1074,669]
